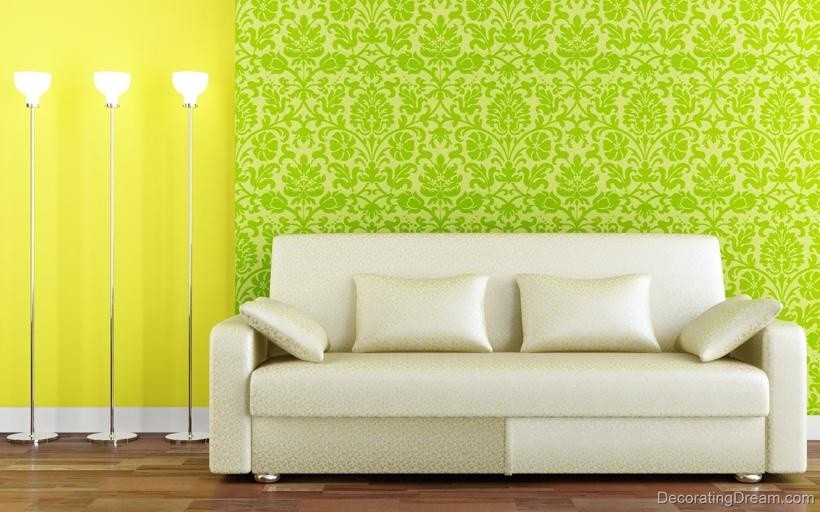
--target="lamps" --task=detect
[5,71,212,445]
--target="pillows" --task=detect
[237,295,329,362]
[515,272,662,354]
[349,270,493,352]
[675,292,783,362]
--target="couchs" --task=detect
[208,229,809,487]
